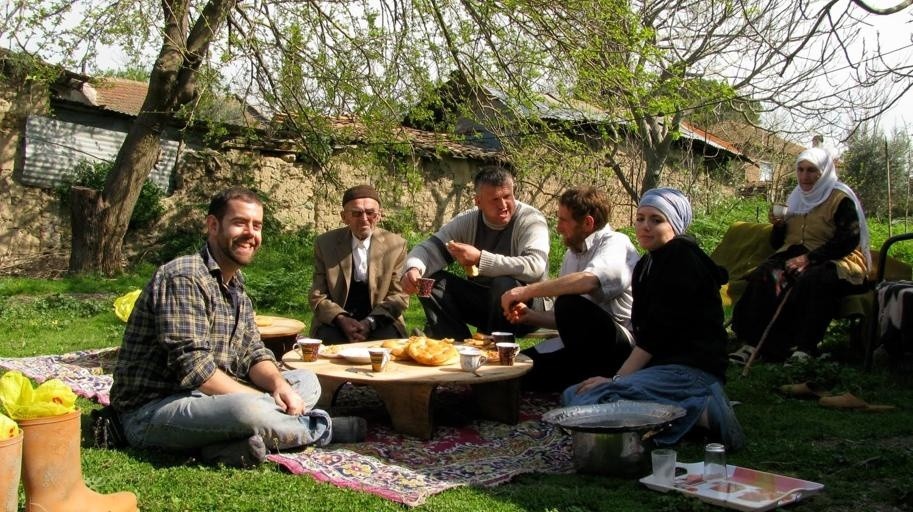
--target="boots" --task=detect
[0,429,23,512]
[17,407,138,512]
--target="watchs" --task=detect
[612,375,624,381]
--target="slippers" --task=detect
[784,350,812,367]
[820,394,895,412]
[778,381,831,398]
[727,345,762,365]
[705,382,745,454]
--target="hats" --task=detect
[343,186,381,207]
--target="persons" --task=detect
[502,186,641,400]
[401,167,550,341]
[726,147,873,364]
[307,185,410,345]
[561,186,747,451]
[108,187,368,466]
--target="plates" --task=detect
[339,348,372,363]
[455,344,483,356]
[318,345,342,359]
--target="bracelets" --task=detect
[366,317,376,332]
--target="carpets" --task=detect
[0,343,652,508]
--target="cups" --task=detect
[416,278,435,297]
[704,443,727,488]
[772,203,789,221]
[292,338,322,361]
[459,351,487,372]
[368,349,390,372]
[491,332,513,346]
[651,449,677,485]
[496,343,520,366]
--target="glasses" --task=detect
[351,211,378,219]
